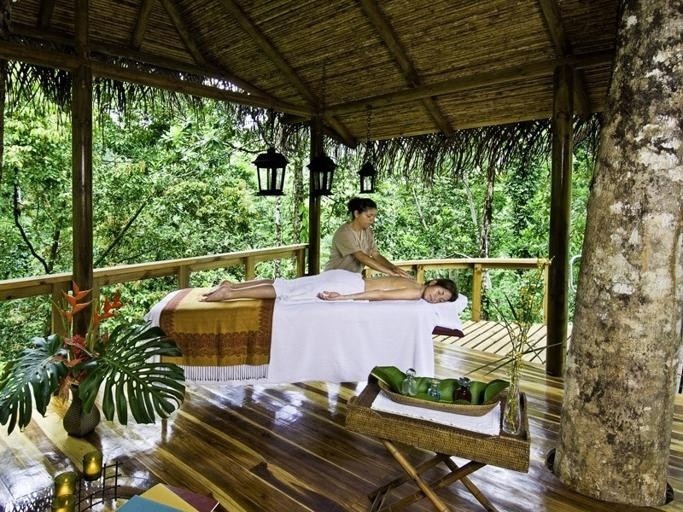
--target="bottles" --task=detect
[401,368,472,405]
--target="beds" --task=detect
[148,285,467,385]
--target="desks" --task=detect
[343,385,530,512]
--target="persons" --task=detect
[323,196,416,280]
[198,267,459,304]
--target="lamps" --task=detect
[247,104,378,197]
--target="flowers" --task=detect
[0,281,186,436]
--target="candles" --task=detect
[50,448,103,512]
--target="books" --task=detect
[114,482,219,511]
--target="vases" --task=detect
[61,384,100,434]
[502,372,522,436]
